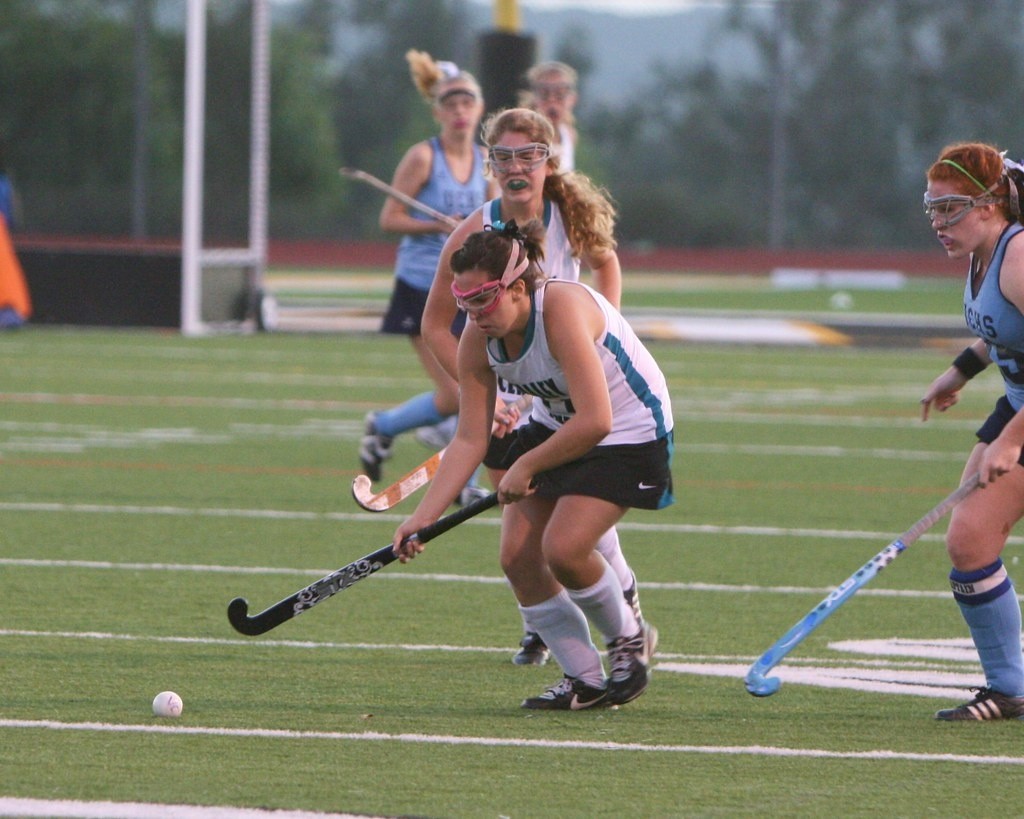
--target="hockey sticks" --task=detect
[338,167,462,230]
[352,392,532,512]
[227,476,541,637]
[741,473,985,697]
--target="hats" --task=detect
[432,77,476,100]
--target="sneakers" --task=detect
[605,616,658,704]
[511,632,550,666]
[359,408,394,481]
[935,684,1024,721]
[521,673,609,711]
[622,568,644,626]
[412,426,449,452]
[455,485,491,506]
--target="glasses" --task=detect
[450,279,507,314]
[488,142,551,171]
[922,191,977,226]
[533,82,574,100]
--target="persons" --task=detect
[392,218,675,711]
[919,144,1024,722]
[360,49,501,508]
[518,61,579,175]
[420,108,659,665]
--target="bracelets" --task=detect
[953,347,987,378]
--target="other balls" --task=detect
[152,690,183,716]
